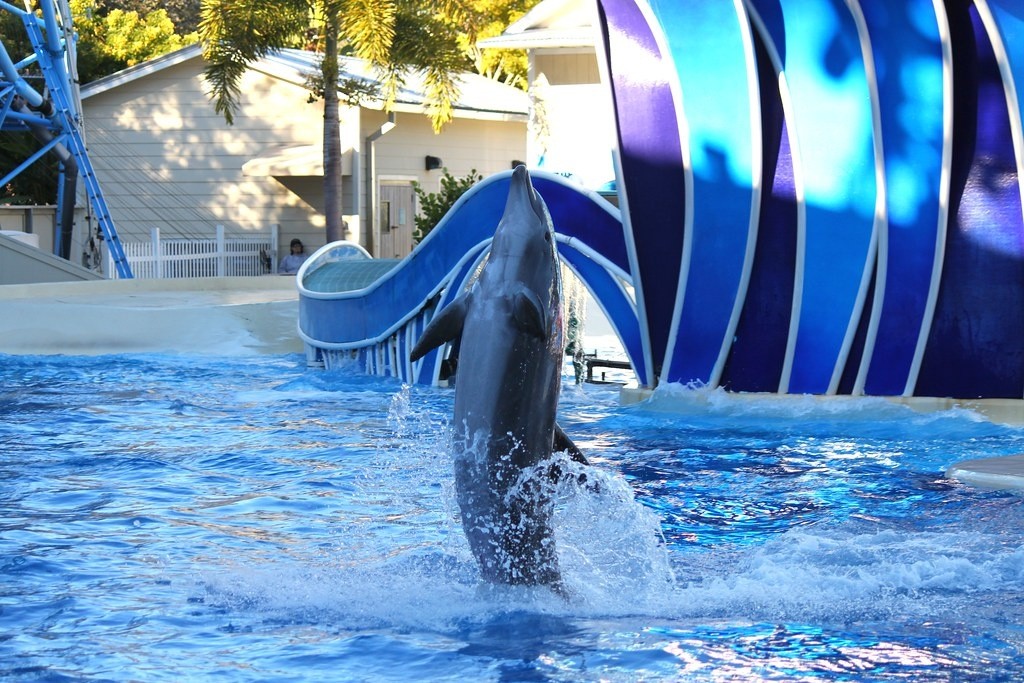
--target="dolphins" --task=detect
[409,164,600,602]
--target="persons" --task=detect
[279,238,308,274]
